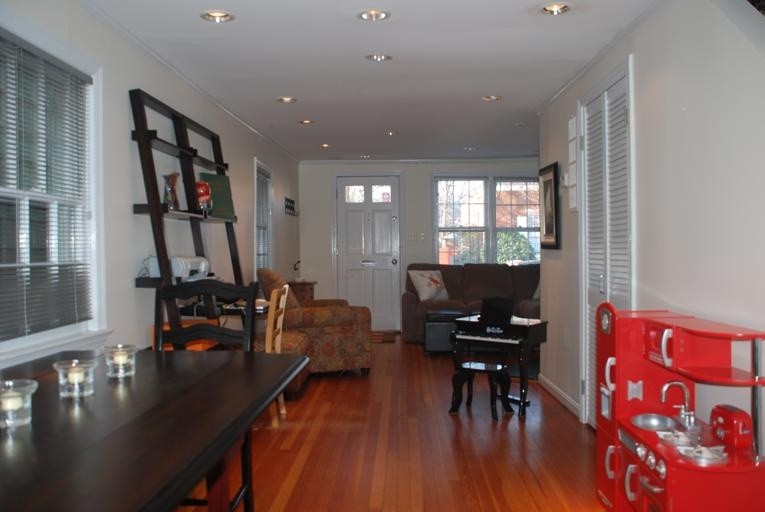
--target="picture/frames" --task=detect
[537,161,561,250]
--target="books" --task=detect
[200,172,235,221]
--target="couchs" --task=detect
[401,261,542,347]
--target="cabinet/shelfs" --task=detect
[289,280,317,301]
[125,88,266,351]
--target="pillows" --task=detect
[408,268,455,303]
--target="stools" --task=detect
[449,361,515,420]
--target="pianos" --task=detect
[449,298,548,415]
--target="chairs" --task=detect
[260,268,375,379]
[154,278,292,430]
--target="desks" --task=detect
[446,313,549,422]
[0,350,310,512]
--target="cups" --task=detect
[102,342,137,378]
[52,359,97,400]
[0,378,39,429]
